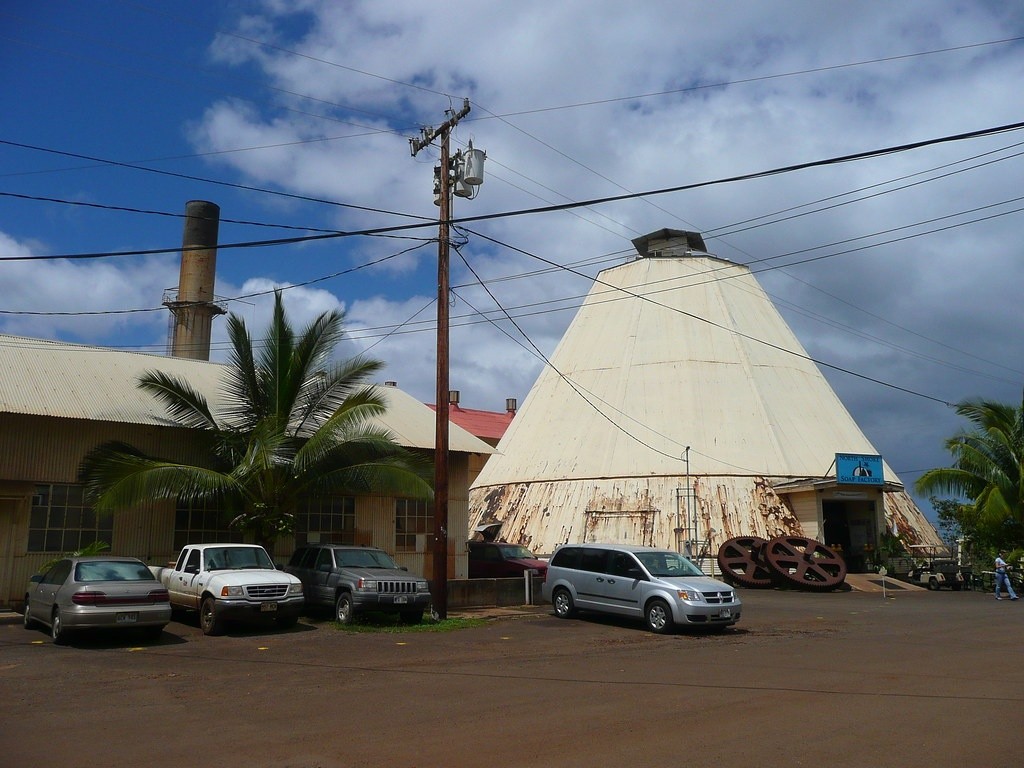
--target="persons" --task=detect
[995,550,1019,600]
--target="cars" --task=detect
[542,542,743,635]
[23,554,173,645]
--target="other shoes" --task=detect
[996,596,1002,600]
[1011,596,1019,600]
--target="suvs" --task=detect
[283,542,432,626]
[468,539,549,578]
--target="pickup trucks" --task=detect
[147,543,305,637]
